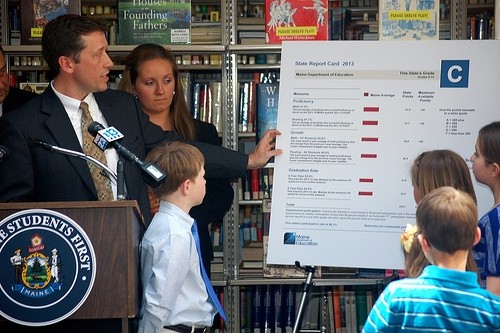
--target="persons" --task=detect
[144,142,228,333]
[0,50,41,118]
[400,150,483,288]
[0,14,280,203]
[361,186,500,333]
[470,121,500,293]
[116,43,234,281]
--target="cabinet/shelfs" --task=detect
[0,1,500,333]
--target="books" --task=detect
[207,219,224,281]
[235,137,277,202]
[8,56,52,95]
[177,70,222,141]
[239,284,390,333]
[235,1,497,66]
[236,68,280,133]
[237,205,408,281]
[8,0,221,46]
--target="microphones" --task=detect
[31,139,127,200]
[88,123,165,187]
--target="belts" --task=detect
[164,324,208,333]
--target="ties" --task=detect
[191,218,226,321]
[79,102,115,201]
